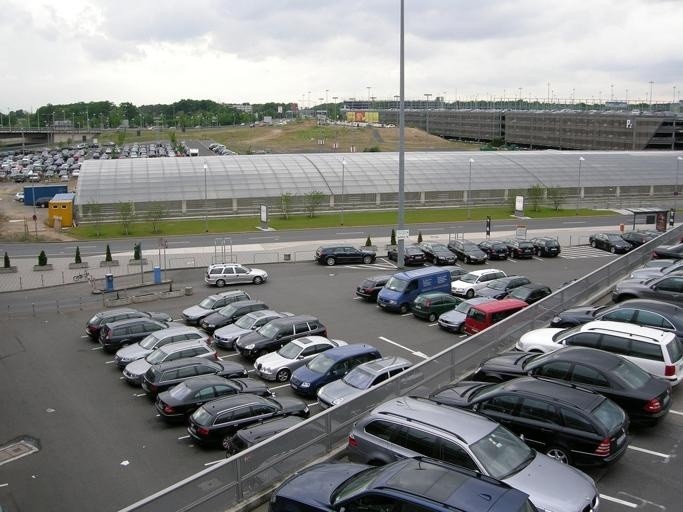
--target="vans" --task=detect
[290,342,382,398]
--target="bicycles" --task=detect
[72,270,89,282]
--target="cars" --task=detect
[549,297,683,344]
[251,335,349,383]
[590,227,683,305]
[211,310,296,352]
[473,346,675,429]
[0,137,239,203]
[155,374,269,426]
[322,119,395,129]
[145,111,292,133]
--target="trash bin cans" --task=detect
[153,266,160,284]
[105,274,113,291]
[620,224,623,233]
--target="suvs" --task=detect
[235,314,327,362]
[182,289,252,326]
[265,454,540,511]
[512,318,683,388]
[200,299,269,335]
[205,263,267,286]
[430,373,632,473]
[83,305,248,397]
[313,243,377,267]
[347,392,604,511]
[356,238,561,337]
[224,413,327,472]
[316,355,423,416]
[186,391,312,450]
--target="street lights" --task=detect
[466,157,475,219]
[424,94,432,132]
[575,157,585,214]
[3,104,145,136]
[393,95,400,125]
[199,159,210,231]
[673,155,682,210]
[299,85,379,118]
[440,77,683,115]
[337,159,347,226]
